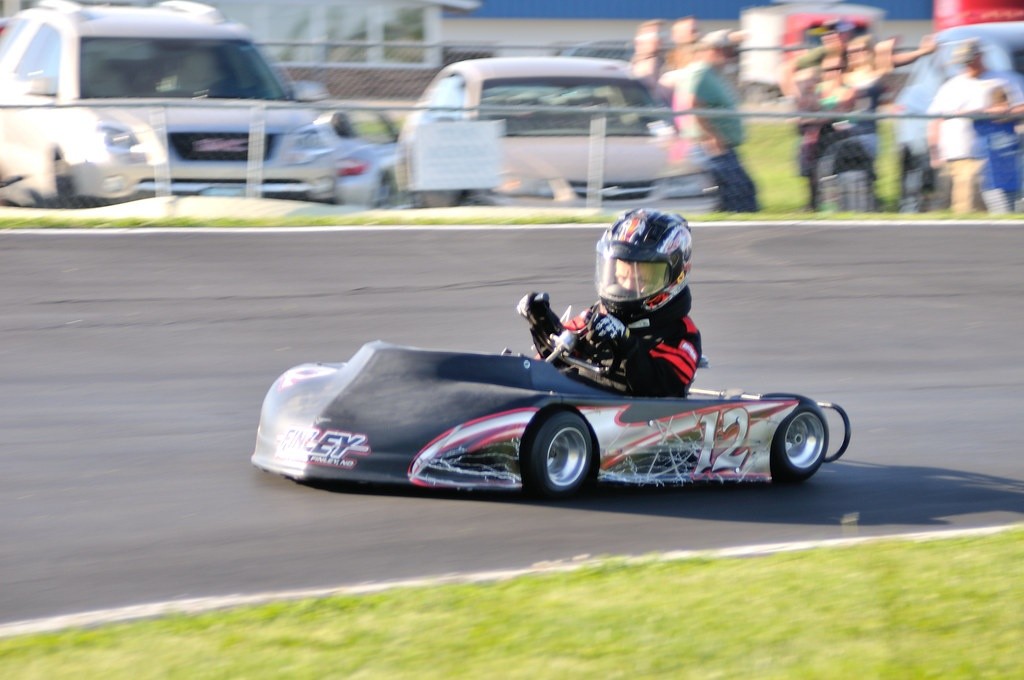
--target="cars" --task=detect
[395,53,720,211]
[895,21,1022,218]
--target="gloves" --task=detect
[586,313,631,351]
[516,290,550,319]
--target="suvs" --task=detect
[1,0,392,205]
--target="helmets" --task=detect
[596,207,695,314]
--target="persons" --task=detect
[629,11,755,212]
[518,210,705,396]
[780,16,1024,210]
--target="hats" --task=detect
[949,39,989,63]
